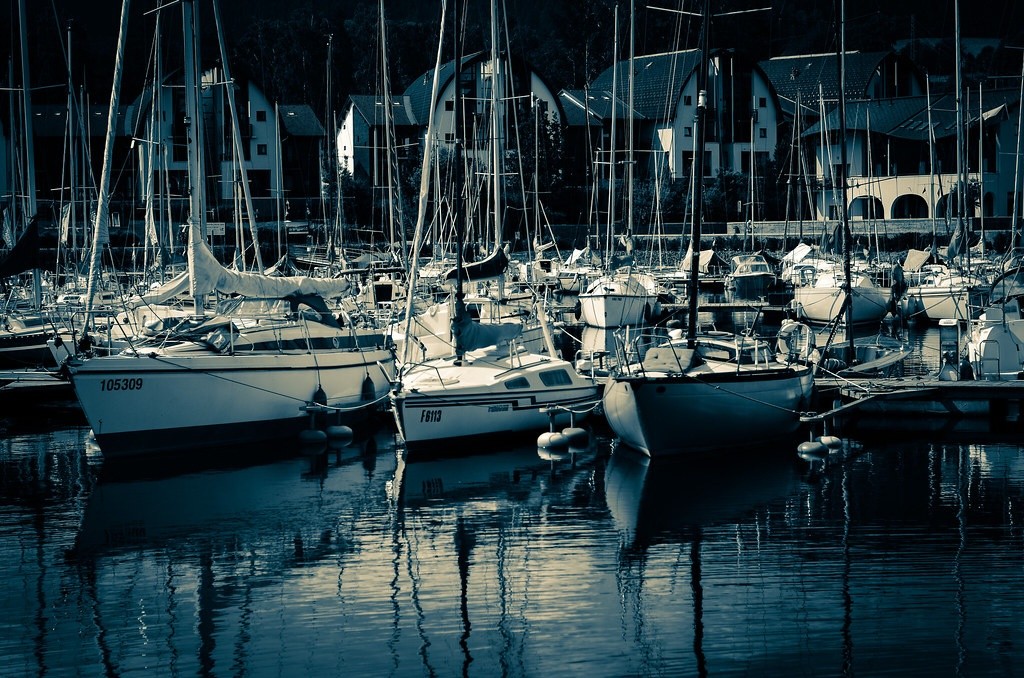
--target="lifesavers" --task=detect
[799,265,816,285]
[778,323,815,358]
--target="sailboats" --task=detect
[0,0,1024,457]
[0,445,1024,677]
